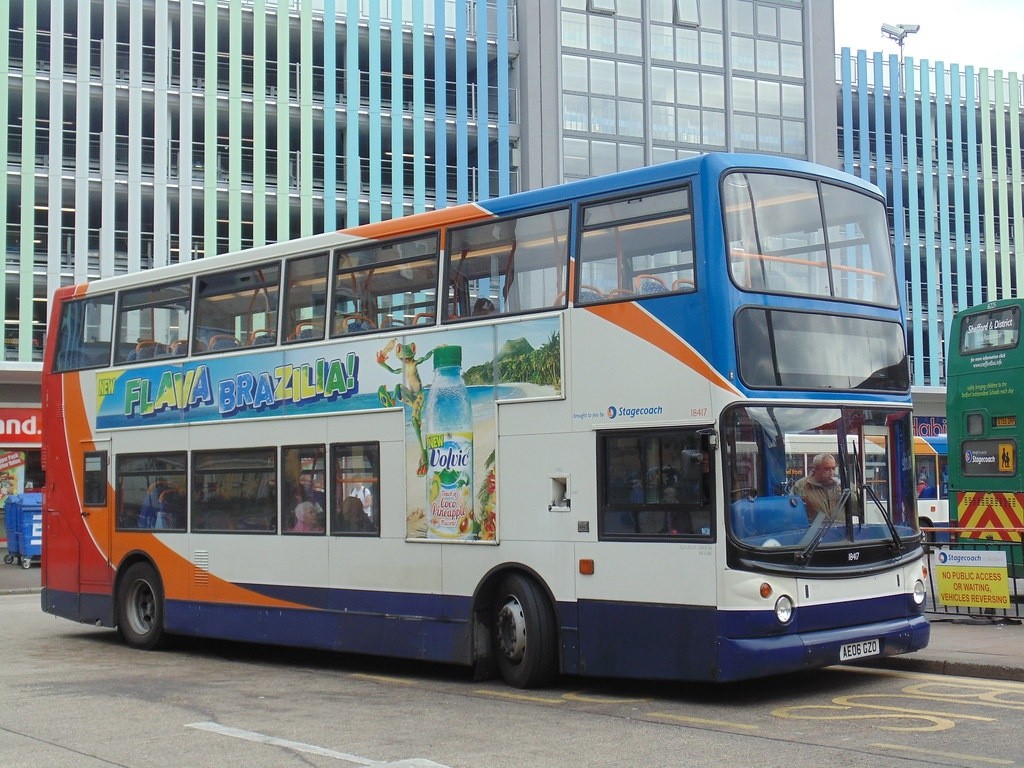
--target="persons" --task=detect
[291,472,372,533]
[790,451,858,523]
[472,298,496,316]
[917,465,948,498]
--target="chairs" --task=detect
[552,273,694,307]
[192,497,300,533]
[125,294,500,362]
[919,486,937,498]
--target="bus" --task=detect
[40,152,940,687]
[728,434,949,554]
[944,297,1024,579]
